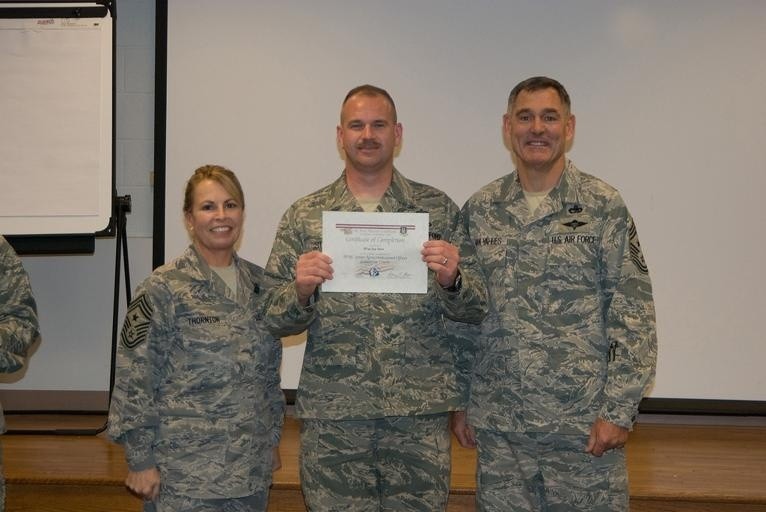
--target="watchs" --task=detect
[442,268,462,291]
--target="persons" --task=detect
[451,77,658,512]
[0,232,40,512]
[106,165,287,512]
[256,85,490,512]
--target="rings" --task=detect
[443,257,448,264]
